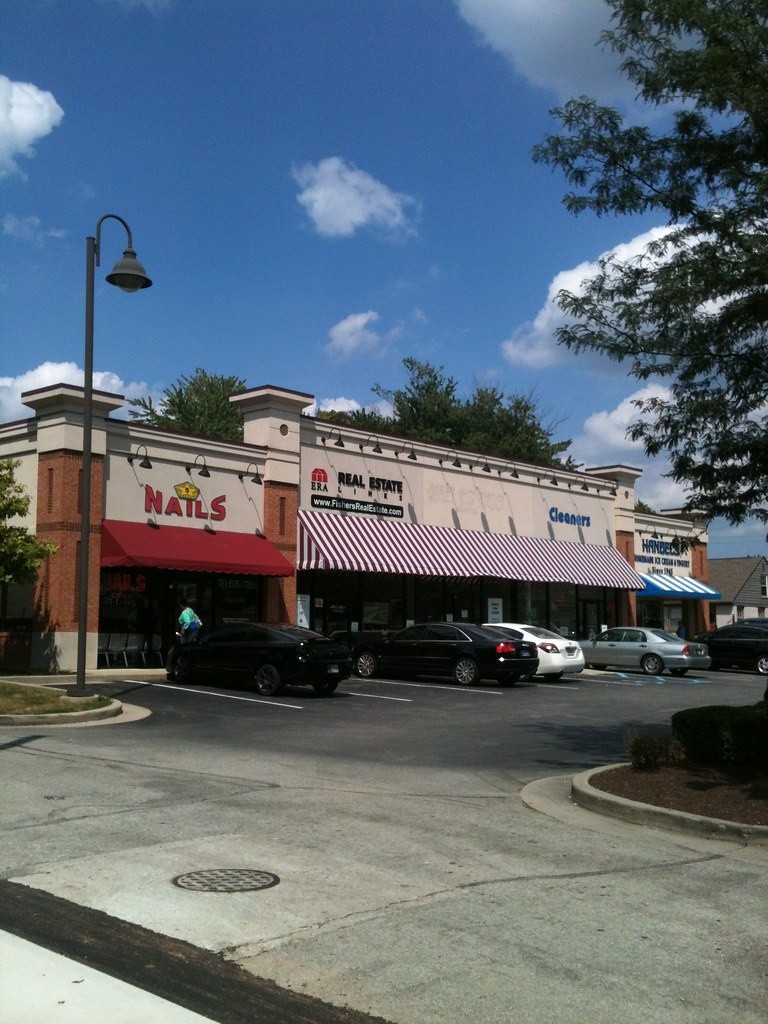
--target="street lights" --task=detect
[68,213,157,696]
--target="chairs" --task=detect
[97,634,111,669]
[145,635,164,666]
[108,634,128,668]
[126,634,147,668]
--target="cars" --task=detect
[686,617,768,675]
[338,625,540,690]
[573,627,712,679]
[475,620,589,682]
[165,617,351,696]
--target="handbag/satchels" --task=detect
[179,621,197,633]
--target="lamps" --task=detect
[359,434,382,454]
[128,444,152,468]
[498,461,520,479]
[640,523,658,539]
[394,441,418,460]
[660,528,680,543]
[685,530,700,547]
[439,450,461,468]
[468,455,492,473]
[535,469,557,486]
[187,453,210,477]
[321,427,344,448]
[239,462,263,486]
[568,475,589,491]
[597,482,617,497]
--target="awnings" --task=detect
[296,510,647,592]
[101,519,295,578]
[636,572,721,599]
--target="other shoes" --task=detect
[167,672,175,680]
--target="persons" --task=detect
[549,620,560,633]
[647,613,661,629]
[676,621,686,640]
[176,598,198,643]
